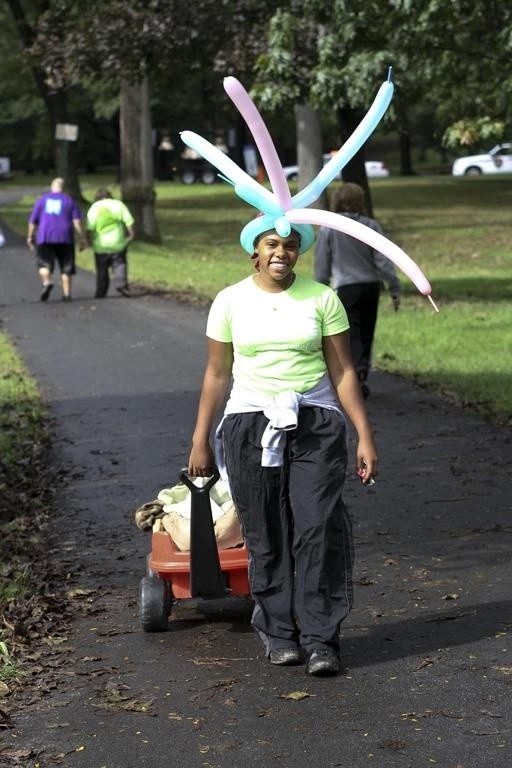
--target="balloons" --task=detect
[178,66,444,313]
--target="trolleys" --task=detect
[134,466,256,632]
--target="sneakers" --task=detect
[116,287,130,298]
[269,647,301,665]
[40,282,54,301]
[307,649,340,676]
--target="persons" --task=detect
[28,177,86,302]
[316,187,400,396]
[190,211,380,676]
[85,188,136,297]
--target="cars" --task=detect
[452,144,511,177]
[281,153,392,181]
